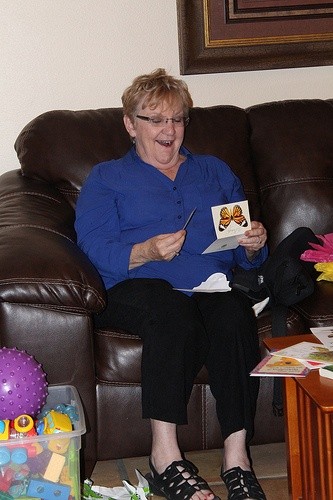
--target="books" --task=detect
[250,326,333,378]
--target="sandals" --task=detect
[220,463,266,500]
[144,452,221,500]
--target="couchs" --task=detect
[0,94,333,458]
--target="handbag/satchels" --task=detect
[233,224,325,304]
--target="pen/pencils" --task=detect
[183,208,197,229]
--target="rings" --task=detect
[174,251,179,256]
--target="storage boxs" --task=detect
[0,385,87,500]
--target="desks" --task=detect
[263,334,333,500]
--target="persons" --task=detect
[74,67,269,500]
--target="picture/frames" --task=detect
[176,0,333,75]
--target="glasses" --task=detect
[136,114,190,128]
